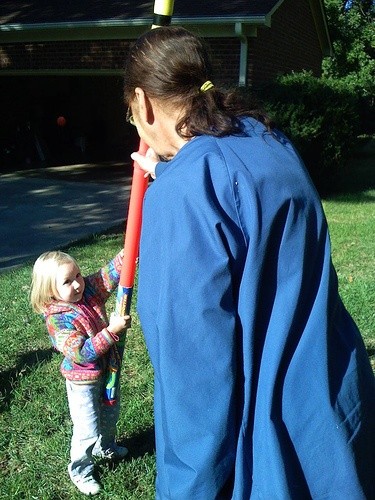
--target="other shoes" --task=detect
[70,475,100,495]
[108,446,128,458]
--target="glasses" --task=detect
[126,103,136,126]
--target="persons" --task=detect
[29,249,140,496]
[121,27,375,500]
